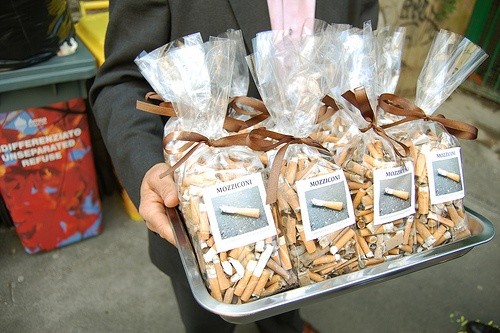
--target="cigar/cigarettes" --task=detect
[162,113,469,303]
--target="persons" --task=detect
[89,0,380,333]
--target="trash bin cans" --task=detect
[76,0,147,222]
[0,37,105,255]
